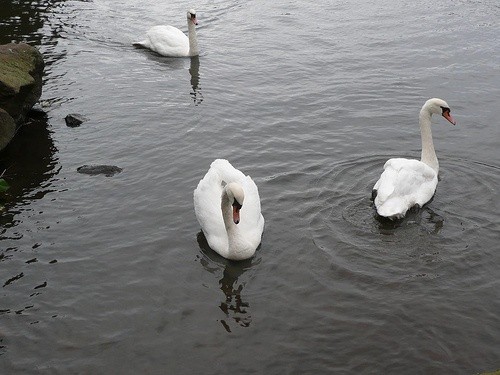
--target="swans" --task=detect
[131,9,199,58]
[193,159,265,261]
[371,98,457,221]
